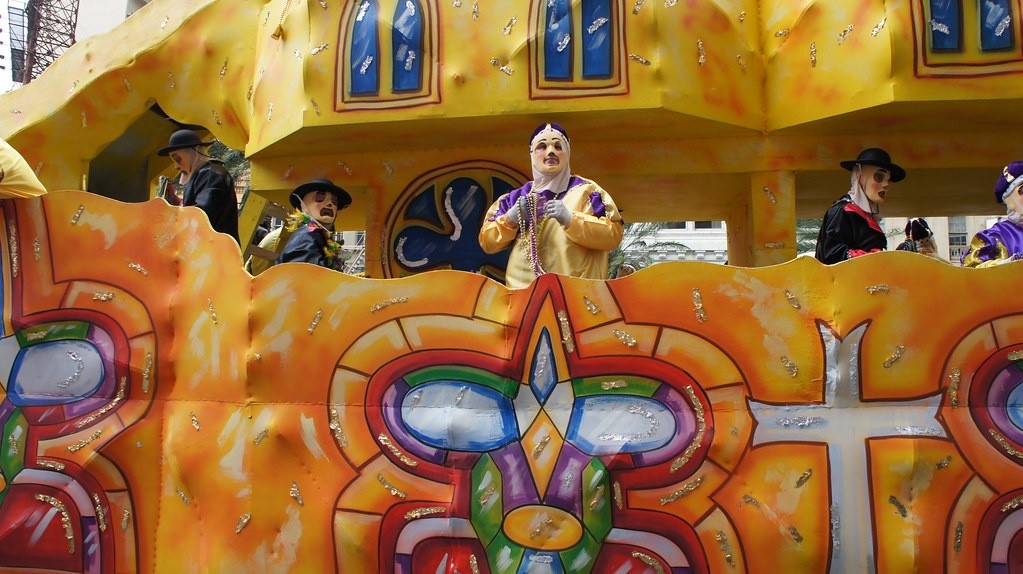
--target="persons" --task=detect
[960,161,1023,268]
[895,218,938,256]
[478,121,623,289]
[158,130,240,246]
[815,148,906,266]
[616,264,636,278]
[275,178,352,272]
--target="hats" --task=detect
[158,129,215,157]
[995,162,1023,204]
[531,122,570,151]
[289,178,352,212]
[840,148,906,183]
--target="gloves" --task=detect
[544,200,573,226]
[507,197,529,223]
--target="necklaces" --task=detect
[516,183,560,276]
